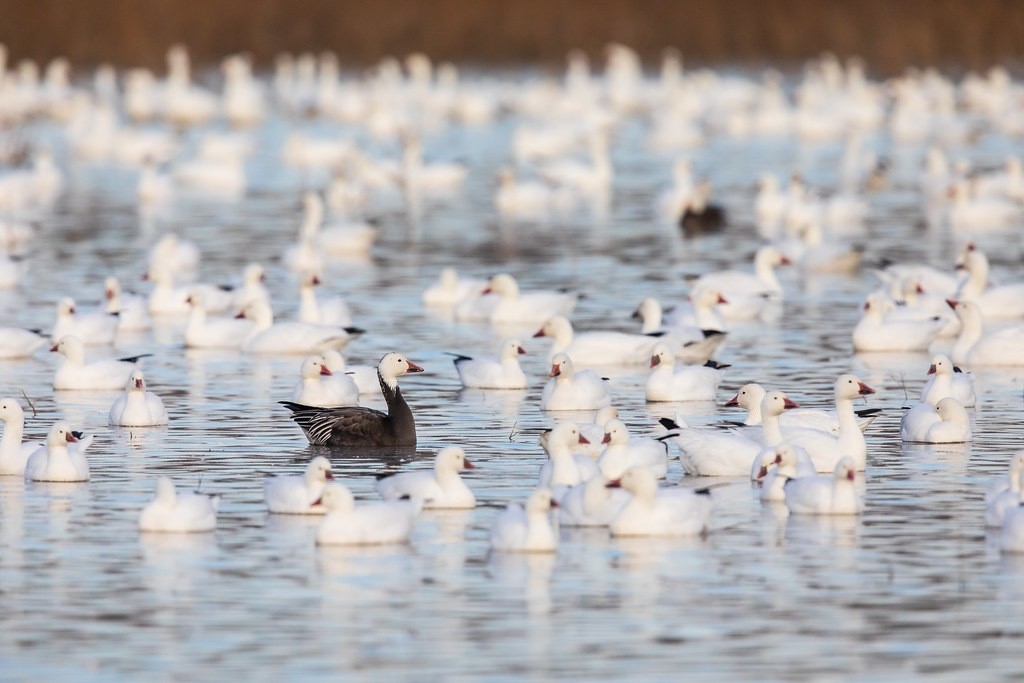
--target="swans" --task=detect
[108,369,170,427]
[422,245,1024,554]
[260,455,334,514]
[136,475,221,533]
[0,189,380,357]
[0,0,1024,240]
[374,446,480,508]
[23,419,94,481]
[274,352,424,459]
[310,482,416,545]
[0,398,47,475]
[49,335,142,391]
